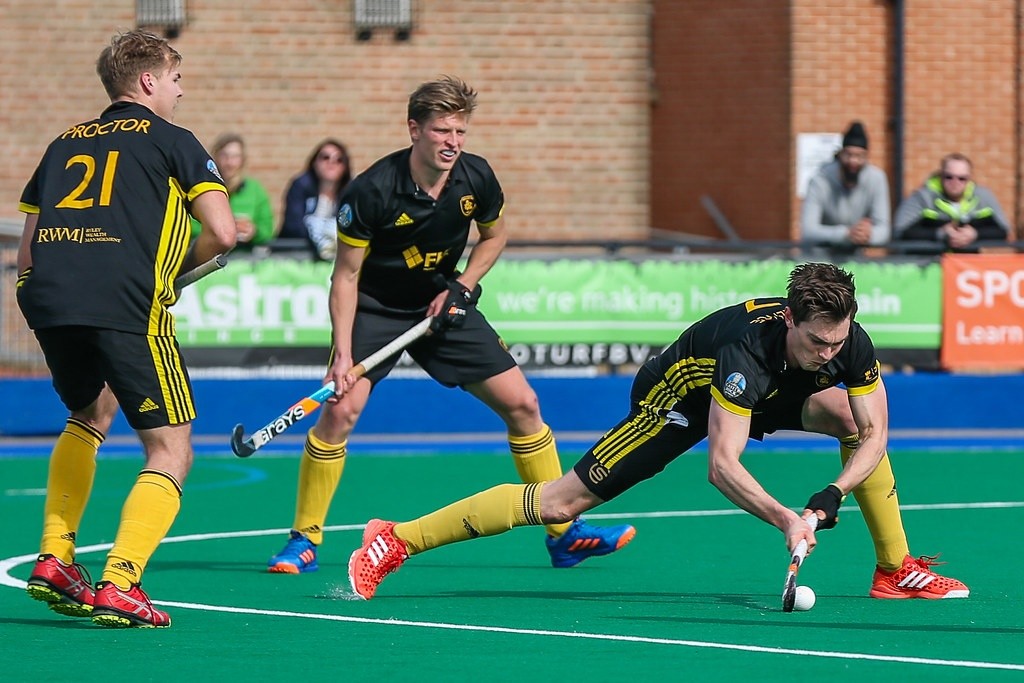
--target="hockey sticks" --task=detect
[232,313,434,458]
[782,513,819,611]
[173,253,227,292]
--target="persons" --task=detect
[267,78,636,574]
[348,261,970,599]
[863,153,1009,255]
[18,29,238,628]
[799,122,892,261]
[188,133,274,255]
[270,139,352,257]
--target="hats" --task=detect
[842,122,868,149]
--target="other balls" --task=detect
[794,586,816,610]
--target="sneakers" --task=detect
[91,580,171,629]
[869,554,970,599]
[26,554,96,617]
[348,518,412,601]
[545,516,637,568]
[266,529,320,573]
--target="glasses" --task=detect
[315,154,348,162]
[938,173,971,182]
